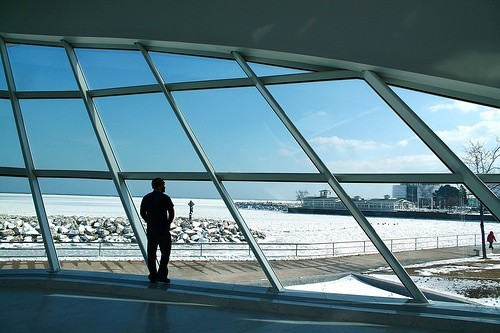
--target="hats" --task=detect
[151,177,166,188]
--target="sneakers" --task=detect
[148,277,170,282]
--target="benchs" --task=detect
[473,248,490,256]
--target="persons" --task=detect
[487,230,497,249]
[140,178,175,283]
